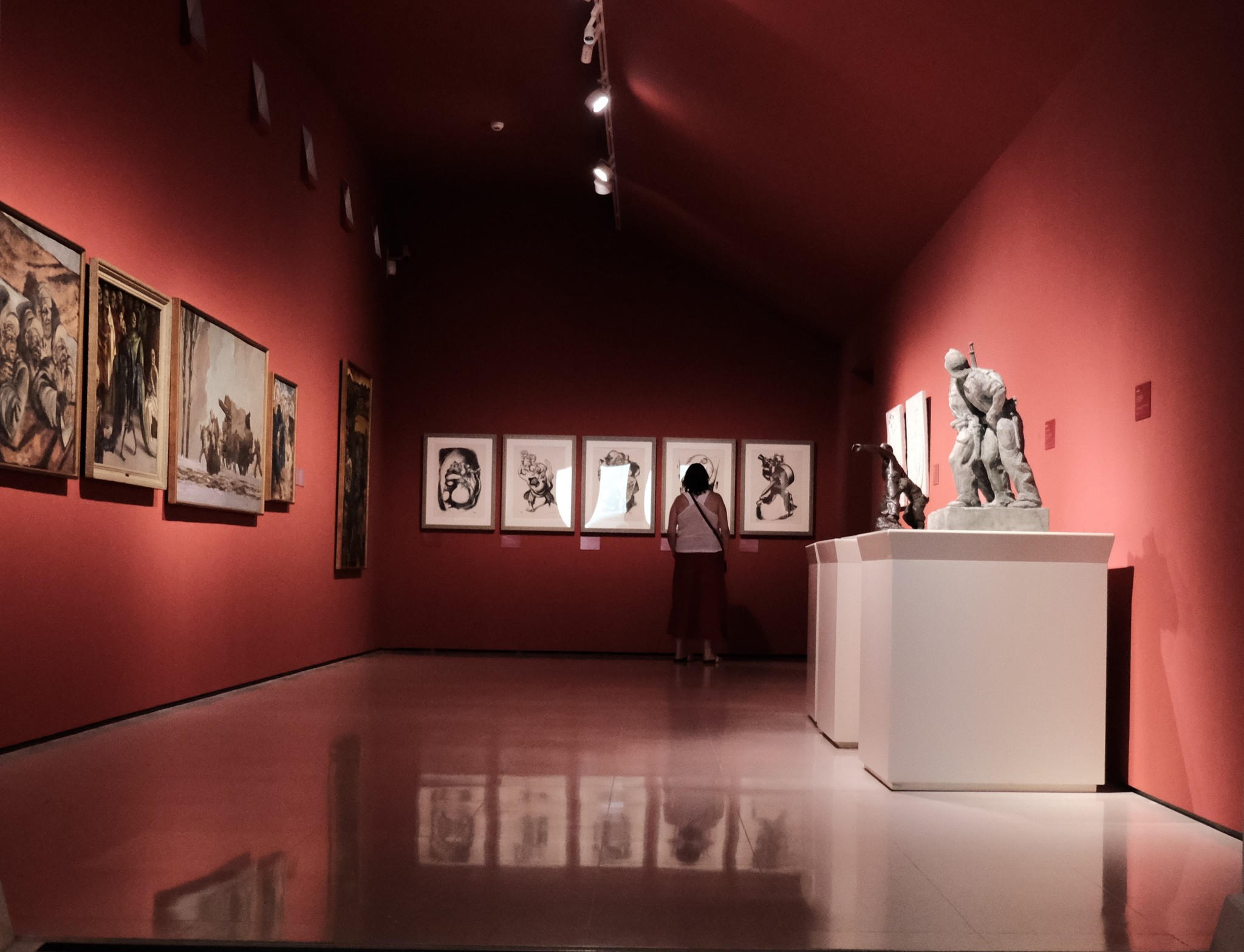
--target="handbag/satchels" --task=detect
[725,561,727,572]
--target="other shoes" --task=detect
[674,658,688,663]
[705,655,721,663]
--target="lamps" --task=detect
[584,83,611,115]
[591,157,615,183]
[581,30,599,64]
[583,5,599,45]
[594,178,614,196]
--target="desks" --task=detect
[802,532,1114,792]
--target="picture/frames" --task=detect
[884,405,903,466]
[264,371,296,504]
[581,436,656,534]
[501,432,577,532]
[421,433,497,530]
[740,438,814,537]
[660,437,736,535]
[904,390,929,499]
[85,256,170,489]
[0,198,86,478]
[168,296,269,516]
[335,356,374,570]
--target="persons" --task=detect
[940,349,1042,508]
[664,464,730,665]
